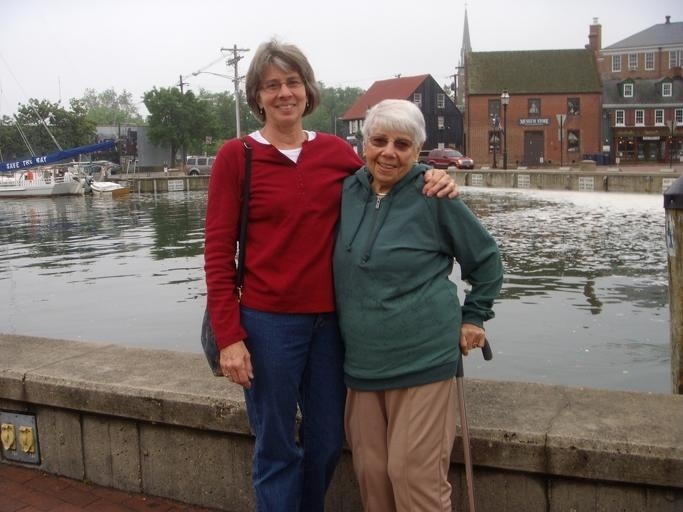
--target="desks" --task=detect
[492,118,497,168]
[191,69,241,139]
[500,88,510,170]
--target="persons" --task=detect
[204,39,460,511]
[331,98,504,512]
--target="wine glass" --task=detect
[225,373,231,377]
[472,342,480,346]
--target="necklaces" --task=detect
[1,49,117,197]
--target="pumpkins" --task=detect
[126,160,140,178]
[125,160,138,195]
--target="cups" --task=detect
[367,135,413,151]
[257,76,304,93]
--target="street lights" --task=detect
[200,284,243,377]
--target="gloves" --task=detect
[90,181,129,197]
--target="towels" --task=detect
[585,155,605,165]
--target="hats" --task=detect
[427,148,474,170]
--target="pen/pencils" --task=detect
[185,155,217,176]
